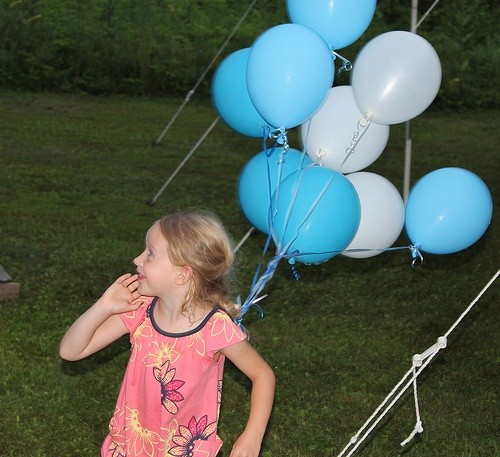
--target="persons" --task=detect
[59,205,275,456]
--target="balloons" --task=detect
[268,167,361,264]
[349,29,443,125]
[282,0,376,61]
[247,23,337,145]
[342,172,403,259]
[406,168,494,254]
[238,152,316,233]
[299,84,390,176]
[212,47,279,138]
